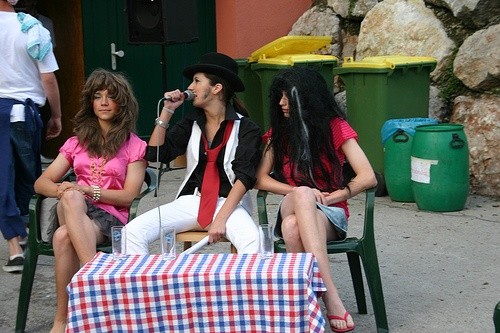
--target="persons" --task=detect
[120,51,265,255]
[255,68,377,332]
[34,70,149,333]
[0,0,62,271]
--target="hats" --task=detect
[182,53,245,92]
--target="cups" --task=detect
[258,223,274,258]
[111,225,126,259]
[160,225,176,261]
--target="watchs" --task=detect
[155,118,169,128]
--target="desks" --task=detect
[65,251,327,333]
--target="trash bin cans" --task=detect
[232,57,264,138]
[246,35,339,137]
[333,55,438,197]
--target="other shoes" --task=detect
[3,255,26,271]
[17,242,26,253]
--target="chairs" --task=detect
[15,166,158,333]
[257,168,388,333]
[172,156,237,254]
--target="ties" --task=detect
[197,120,234,229]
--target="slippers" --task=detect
[325,310,355,332]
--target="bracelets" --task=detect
[163,107,174,114]
[91,185,101,202]
[346,185,352,197]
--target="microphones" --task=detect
[163,90,193,101]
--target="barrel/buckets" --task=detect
[410,123,470,212]
[382,118,439,202]
